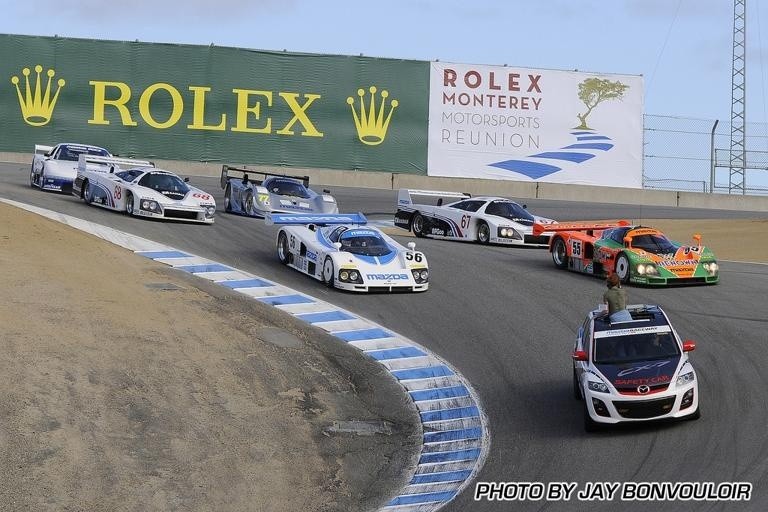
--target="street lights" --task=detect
[710,120,719,193]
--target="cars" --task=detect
[533,219,720,285]
[572,304,698,424]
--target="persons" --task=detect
[643,333,669,354]
[603,274,632,323]
